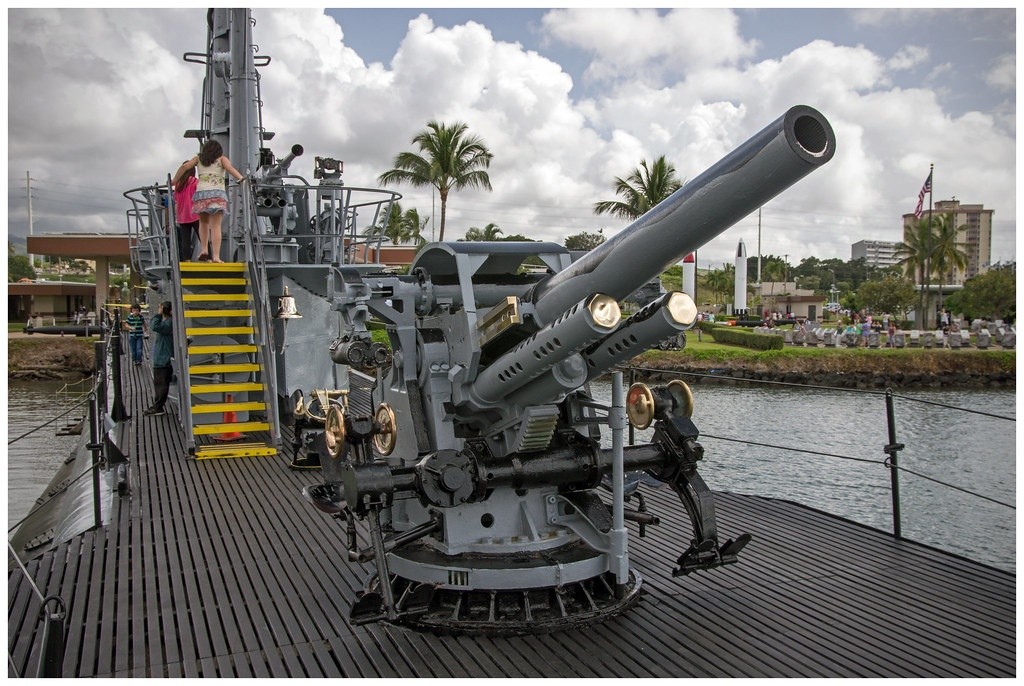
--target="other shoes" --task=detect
[144,408,164,415]
[134,361,142,366]
[198,252,209,262]
[213,259,224,264]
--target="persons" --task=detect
[762,309,896,348]
[951,321,960,332]
[160,190,174,208]
[697,310,715,323]
[171,139,244,263]
[976,317,988,333]
[125,303,148,366]
[940,307,949,334]
[142,300,174,416]
[73,312,77,325]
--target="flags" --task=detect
[915,172,931,219]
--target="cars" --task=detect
[735,311,808,329]
